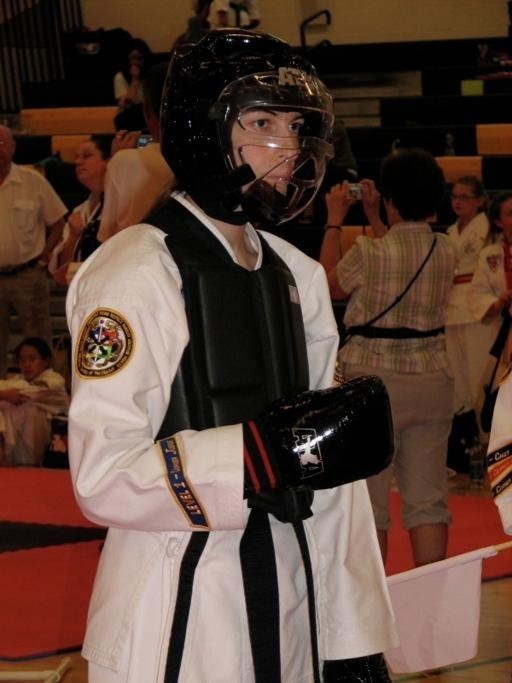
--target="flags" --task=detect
[385,544,500,674]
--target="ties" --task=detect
[0,254,40,278]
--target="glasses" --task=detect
[450,192,481,202]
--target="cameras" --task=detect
[347,185,365,201]
[137,135,152,148]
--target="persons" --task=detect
[0,0,512,467]
[61,27,399,683]
[316,146,460,682]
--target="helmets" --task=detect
[157,25,338,229]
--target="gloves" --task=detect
[243,373,397,499]
